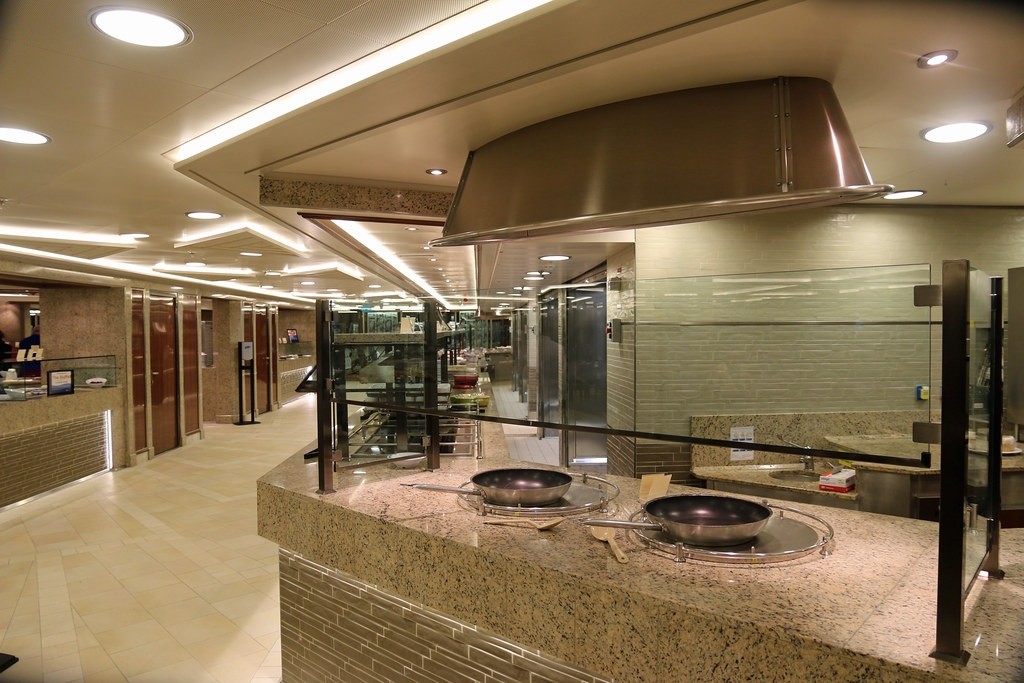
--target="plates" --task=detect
[968,447,1023,456]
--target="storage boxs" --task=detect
[370,381,451,412]
[819,465,858,493]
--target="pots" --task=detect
[400,468,573,506]
[583,494,772,547]
[449,394,490,409]
[453,374,479,389]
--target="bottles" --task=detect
[6,368,17,380]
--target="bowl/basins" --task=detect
[85,378,107,388]
[3,388,25,400]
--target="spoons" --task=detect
[590,525,629,564]
[482,517,566,531]
